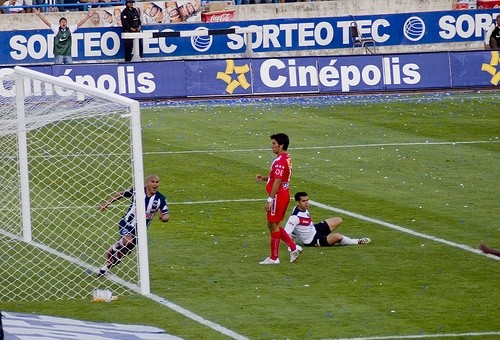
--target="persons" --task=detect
[32,7,95,64]
[484,15,500,50]
[255,133,304,265]
[1,0,25,14]
[97,174,170,279]
[479,243,500,257]
[284,192,371,248]
[121,0,143,61]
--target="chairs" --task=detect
[350,22,376,54]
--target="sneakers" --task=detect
[288,244,304,262]
[260,257,281,265]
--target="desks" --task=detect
[201,9,236,23]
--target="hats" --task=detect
[126,0,135,3]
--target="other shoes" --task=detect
[358,237,371,244]
